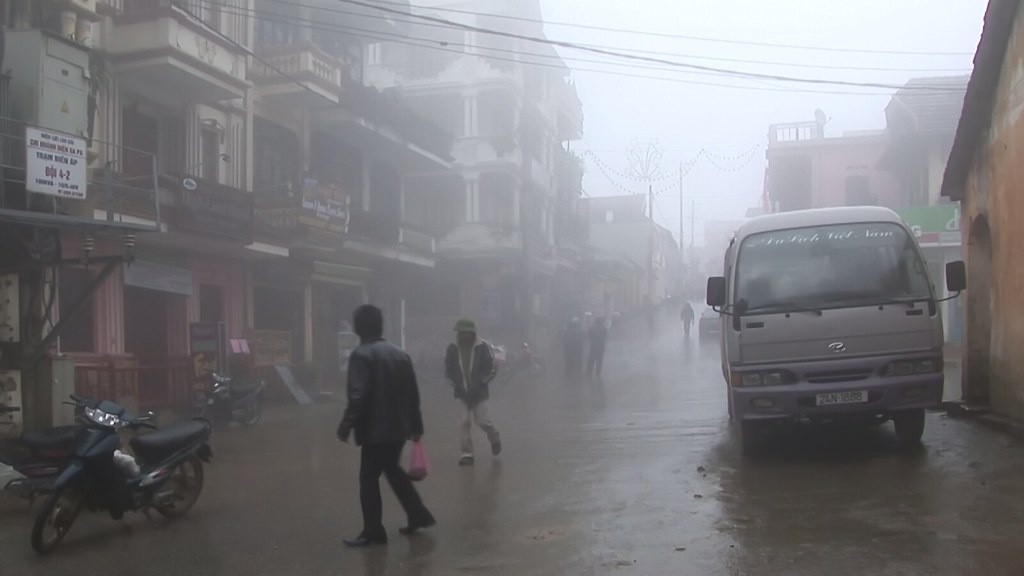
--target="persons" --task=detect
[445,320,501,465]
[563,315,608,378]
[681,303,694,337]
[337,304,437,547]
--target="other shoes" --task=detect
[458,453,474,465]
[491,442,501,455]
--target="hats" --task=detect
[453,319,476,334]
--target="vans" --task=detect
[704,205,969,465]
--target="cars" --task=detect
[698,307,722,333]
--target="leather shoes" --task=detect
[399,518,435,532]
[343,527,389,547]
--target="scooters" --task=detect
[200,368,266,431]
[0,401,92,510]
[29,393,216,558]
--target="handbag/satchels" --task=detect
[409,439,427,481]
